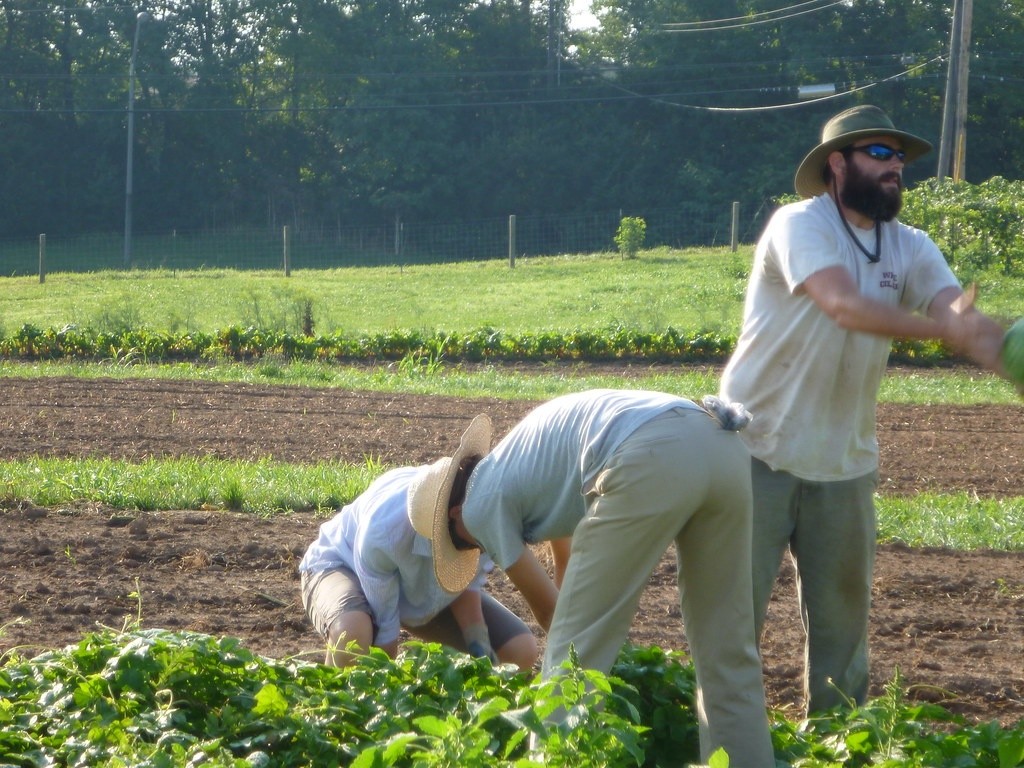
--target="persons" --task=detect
[406,387,776,768]
[298,464,539,678]
[719,105,1007,719]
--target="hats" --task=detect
[795,104,932,198]
[408,413,492,595]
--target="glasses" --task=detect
[837,143,908,162]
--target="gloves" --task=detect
[462,625,498,668]
